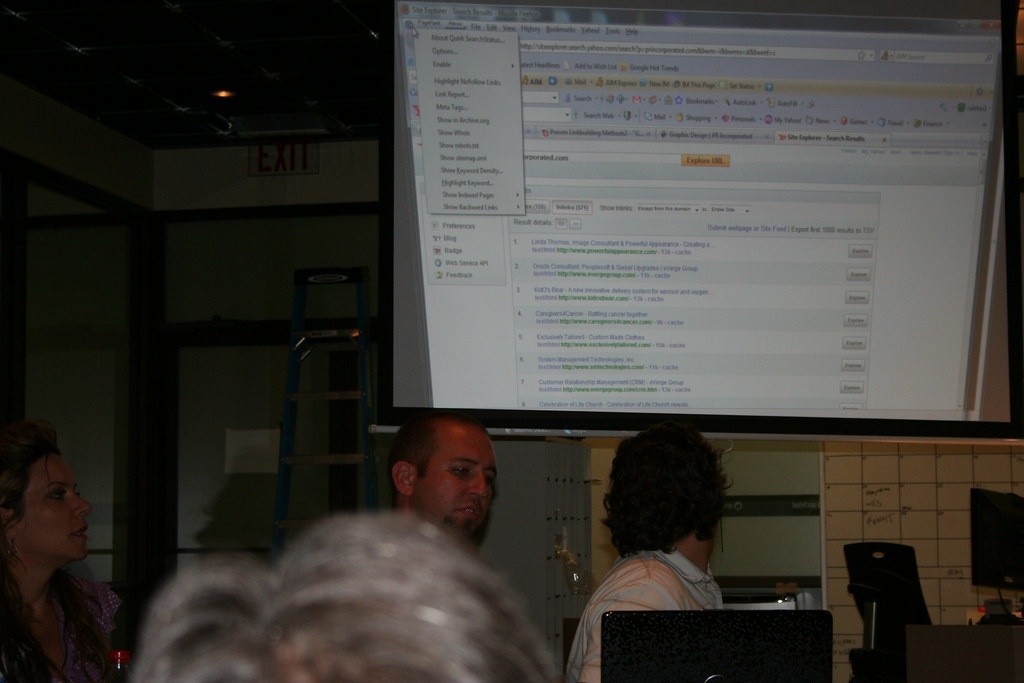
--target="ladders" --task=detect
[264,265,379,576]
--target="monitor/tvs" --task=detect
[970,487,1024,591]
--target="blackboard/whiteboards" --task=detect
[818,442,1023,683]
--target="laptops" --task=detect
[602,609,835,683]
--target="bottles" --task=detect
[973,607,985,626]
[96,650,133,683]
[783,583,798,610]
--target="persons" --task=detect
[0,419,121,683]
[565,421,731,683]
[129,509,561,683]
[388,409,498,544]
